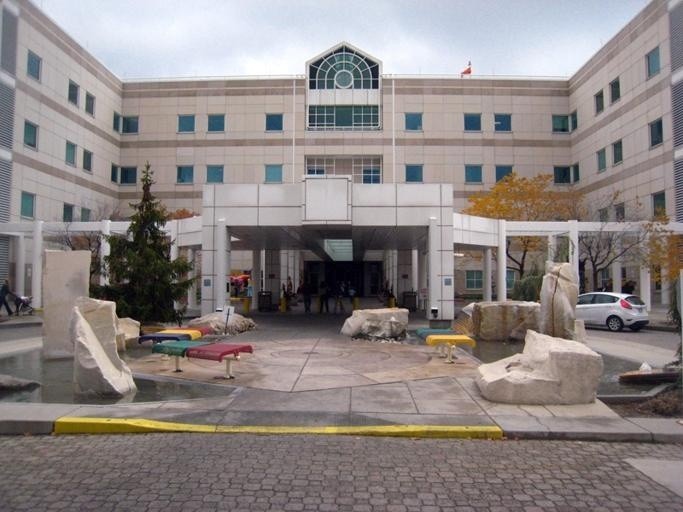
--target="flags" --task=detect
[461,68,471,75]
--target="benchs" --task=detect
[416,327,475,363]
[152,340,254,379]
[138,327,210,346]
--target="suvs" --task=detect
[575,292,648,332]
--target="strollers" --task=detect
[10,294,34,316]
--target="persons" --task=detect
[0,277,12,317]
[281,275,357,314]
[245,277,252,297]
[595,278,637,295]
[232,279,242,297]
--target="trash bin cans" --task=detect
[257,291,272,312]
[403,291,417,313]
